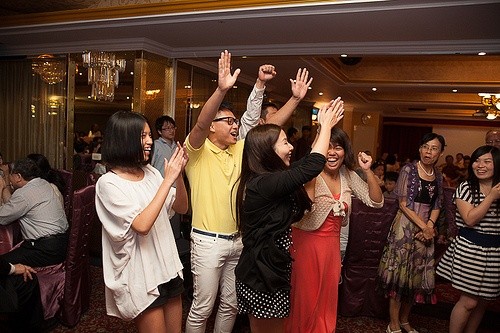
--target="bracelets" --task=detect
[427,219,434,225]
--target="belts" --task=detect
[193,228,240,240]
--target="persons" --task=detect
[248,126,384,333]
[151,117,185,239]
[485,131,500,151]
[0,154,70,333]
[95,110,189,333]
[183,49,313,333]
[234,97,345,333]
[376,133,445,333]
[354,152,471,196]
[434,145,500,333]
[73,132,102,170]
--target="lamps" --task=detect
[30,54,79,85]
[81,51,127,102]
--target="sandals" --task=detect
[400,322,419,333]
[386,325,401,333]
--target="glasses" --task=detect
[162,127,176,130]
[212,117,239,125]
[421,146,439,153]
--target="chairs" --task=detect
[32,184,99,330]
[58,167,77,220]
[337,196,400,322]
[434,186,463,304]
